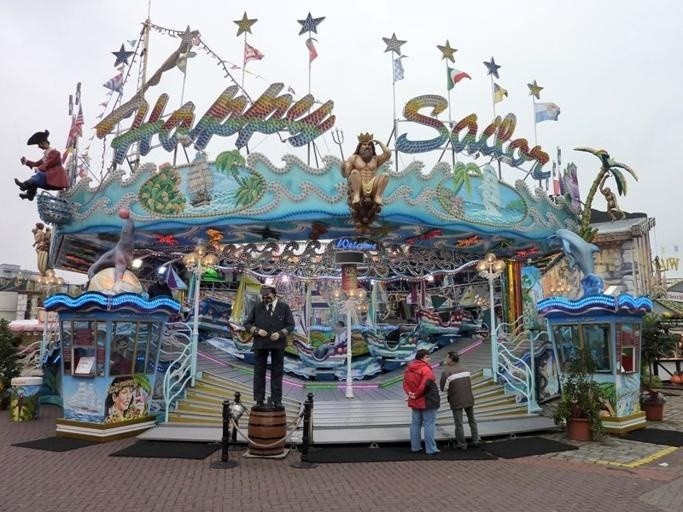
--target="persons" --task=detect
[317,318,347,360]
[403,348,442,456]
[147,274,174,301]
[15,129,70,201]
[341,133,390,207]
[243,284,295,409]
[12,337,33,355]
[439,352,481,450]
[104,377,135,424]
[382,294,399,321]
[32,223,46,277]
[46,228,51,248]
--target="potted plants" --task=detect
[552,343,622,442]
[641,311,673,422]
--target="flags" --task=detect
[244,42,264,65]
[305,38,318,62]
[534,102,560,122]
[60,146,73,164]
[392,55,407,83]
[175,52,197,73]
[75,105,83,124]
[446,67,472,90]
[103,72,124,96]
[68,125,82,139]
[492,81,508,105]
